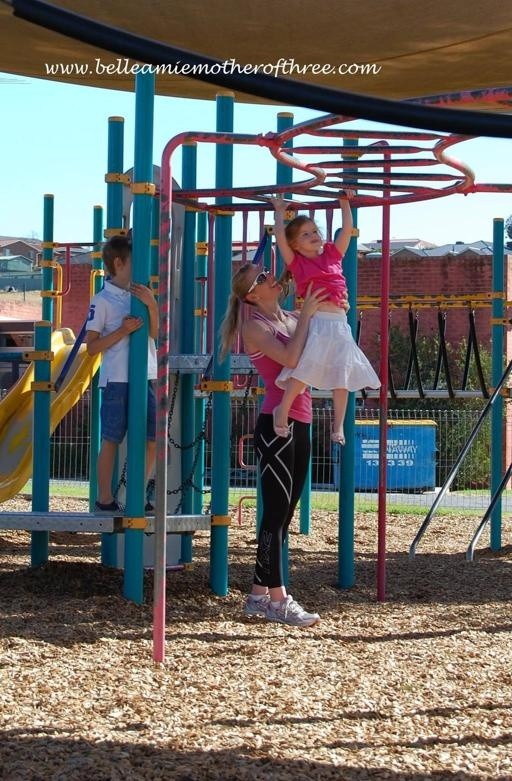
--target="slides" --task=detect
[0,329,102,506]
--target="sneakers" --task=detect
[243,596,271,615]
[144,504,156,516]
[266,595,320,626]
[94,500,125,516]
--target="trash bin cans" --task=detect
[331,419,442,496]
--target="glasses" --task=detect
[247,267,270,293]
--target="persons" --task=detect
[85,235,159,511]
[264,188,357,447]
[218,262,351,627]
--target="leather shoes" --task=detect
[273,406,289,438]
[329,422,346,447]
